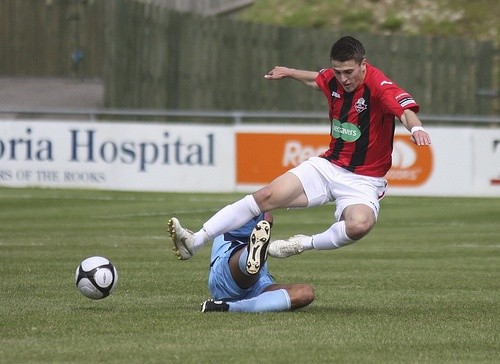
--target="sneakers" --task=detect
[267,234,310,259]
[167,217,195,261]
[245,220,271,274]
[201,299,229,313]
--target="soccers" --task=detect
[74,256,118,300]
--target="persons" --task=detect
[200,209,316,313]
[167,35,430,261]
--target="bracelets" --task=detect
[411,127,427,135]
